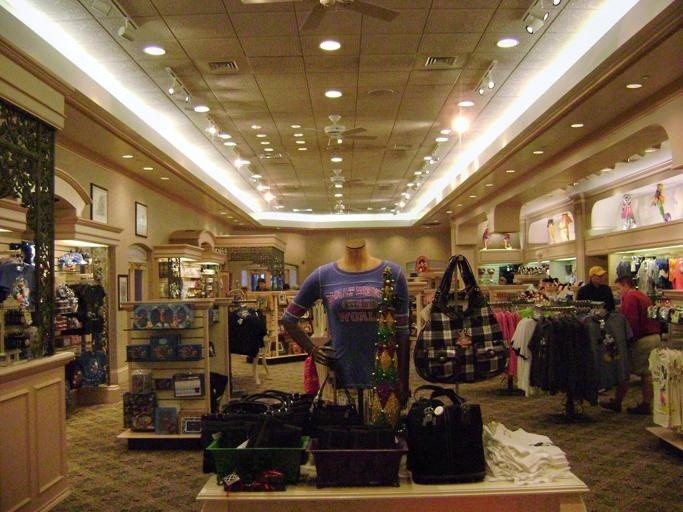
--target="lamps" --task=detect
[522,15,546,34]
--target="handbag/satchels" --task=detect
[413,255,508,383]
[406,385,487,484]
[301,348,322,395]
[312,376,362,429]
[315,420,402,487]
[198,388,311,452]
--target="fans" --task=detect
[298,113,377,150]
[315,169,362,190]
[242,0,400,35]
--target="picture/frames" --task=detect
[135,202,149,237]
[91,183,108,222]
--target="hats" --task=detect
[589,266,607,278]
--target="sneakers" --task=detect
[598,397,623,413]
[627,401,652,417]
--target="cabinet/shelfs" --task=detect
[116,299,230,439]
[152,243,228,301]
[230,290,324,360]
[407,279,434,338]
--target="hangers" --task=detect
[578,300,607,312]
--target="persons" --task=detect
[598,276,661,416]
[279,238,414,426]
[560,214,573,242]
[619,193,636,229]
[256,278,269,291]
[482,229,492,249]
[576,266,615,323]
[501,234,513,248]
